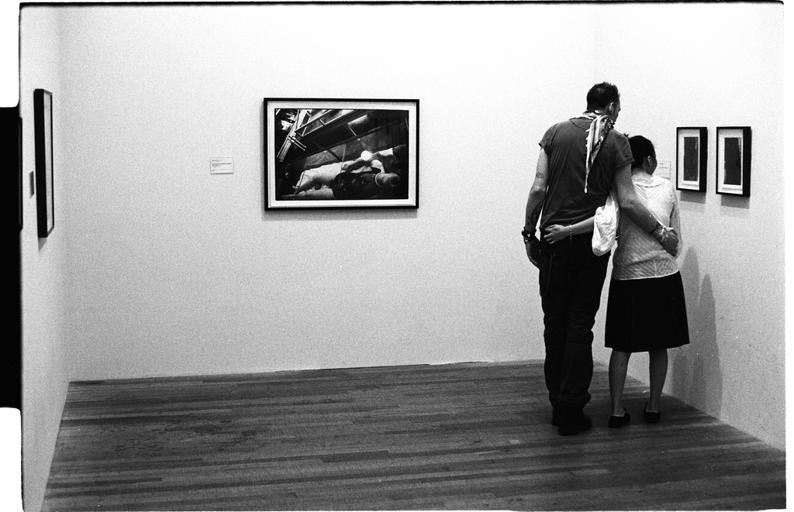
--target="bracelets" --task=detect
[568,224,573,239]
[520,228,537,241]
[651,224,670,243]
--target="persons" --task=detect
[290,149,399,200]
[520,82,679,435]
[540,135,690,431]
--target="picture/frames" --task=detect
[262,98,420,211]
[34,88,55,238]
[716,126,752,197]
[675,126,708,194]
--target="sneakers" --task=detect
[553,408,592,435]
[643,405,661,423]
[609,412,630,428]
